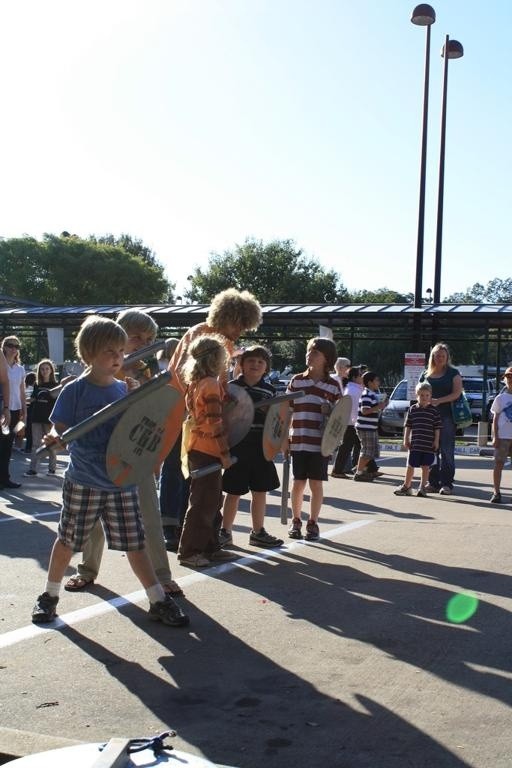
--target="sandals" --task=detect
[162,581,183,597]
[64,576,94,592]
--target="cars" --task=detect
[375,379,502,436]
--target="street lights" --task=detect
[432,34,464,349]
[405,3,436,353]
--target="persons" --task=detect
[33,291,342,623]
[417,344,463,495]
[393,381,444,498]
[489,367,511,503]
[0,336,59,489]
[486,399,495,441]
[329,358,389,482]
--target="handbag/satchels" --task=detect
[451,389,472,424]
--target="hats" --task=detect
[503,366,512,377]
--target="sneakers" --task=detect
[491,492,501,502]
[1,480,21,489]
[394,484,413,496]
[305,520,319,540]
[331,463,384,481]
[32,592,59,623]
[164,527,236,567]
[148,594,190,627]
[249,528,284,547]
[287,517,302,538]
[417,483,452,497]
[14,446,55,477]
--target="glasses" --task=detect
[4,342,20,350]
